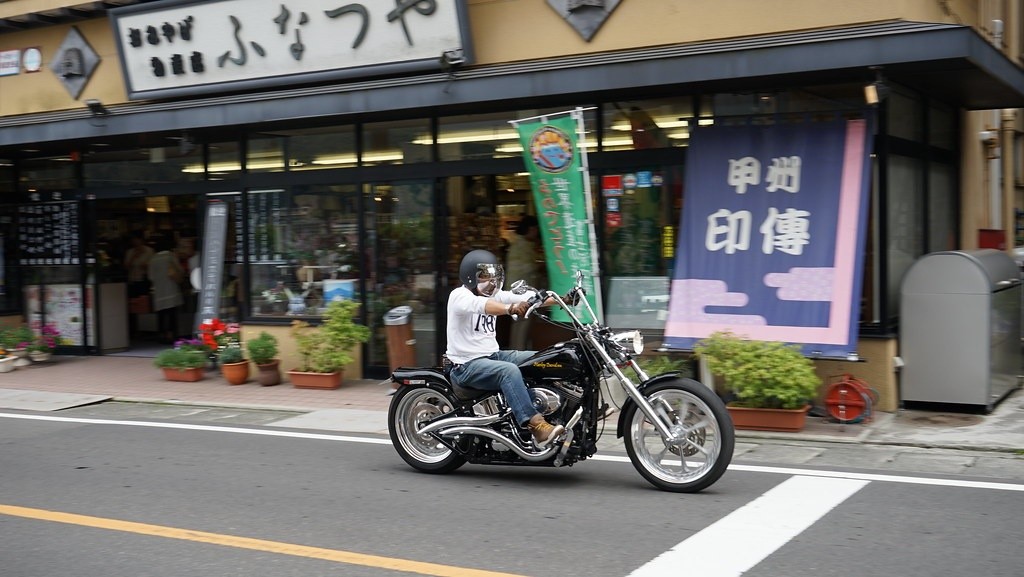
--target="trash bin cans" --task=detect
[382,305,416,387]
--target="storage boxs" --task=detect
[325,278,362,307]
[138,314,159,331]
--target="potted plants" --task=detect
[220,346,250,384]
[287,300,372,390]
[248,331,282,386]
[694,331,814,431]
[152,350,207,382]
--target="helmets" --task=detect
[458,250,504,297]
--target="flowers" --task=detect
[176,317,241,361]
[0,316,76,359]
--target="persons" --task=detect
[445,249,586,447]
[506,216,540,350]
[119,231,200,343]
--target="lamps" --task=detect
[440,48,465,92]
[85,99,108,128]
[864,67,891,106]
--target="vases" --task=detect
[29,351,50,362]
[0,356,19,373]
[7,348,32,366]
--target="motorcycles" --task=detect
[380,268,736,494]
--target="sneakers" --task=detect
[529,414,564,447]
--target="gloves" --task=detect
[562,287,587,307]
[507,301,530,320]
